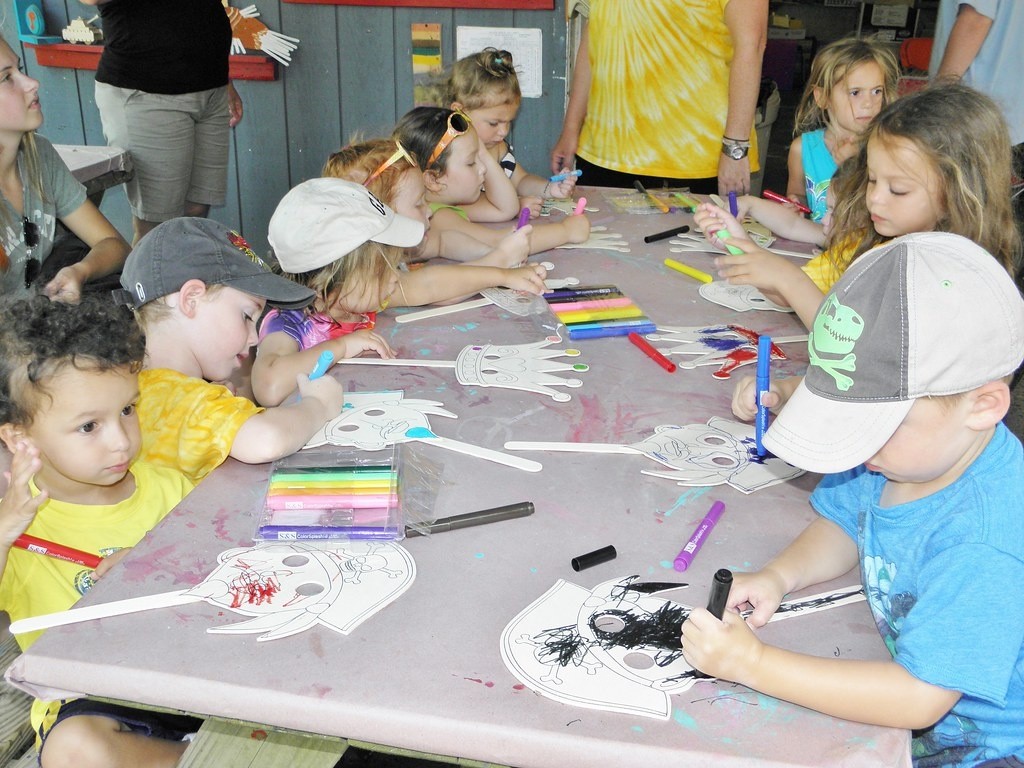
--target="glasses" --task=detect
[423,107,471,171]
[361,139,417,186]
[22,216,41,287]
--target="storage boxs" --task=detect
[768,27,806,40]
[759,39,797,91]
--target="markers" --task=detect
[715,227,742,255]
[756,334,771,463]
[541,285,656,341]
[405,502,536,539]
[627,331,676,373]
[728,191,738,218]
[665,258,713,284]
[258,525,398,540]
[266,466,399,509]
[548,169,582,182]
[645,225,690,244]
[516,208,531,229]
[573,197,587,216]
[674,500,727,573]
[633,179,696,214]
[763,189,813,213]
[297,351,334,401]
[693,568,734,677]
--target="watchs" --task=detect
[722,140,748,160]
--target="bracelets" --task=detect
[723,136,749,142]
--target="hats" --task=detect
[762,230,1023,474]
[268,177,424,273]
[112,217,316,309]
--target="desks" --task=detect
[52,143,133,210]
[5,187,912,768]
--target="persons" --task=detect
[723,156,858,248]
[1,295,204,768]
[929,2,1024,224]
[694,86,1024,332]
[81,1,242,248]
[322,46,590,306]
[782,38,901,223]
[0,33,132,309]
[251,177,554,408]
[681,232,1024,768]
[551,0,769,196]
[120,216,344,484]
